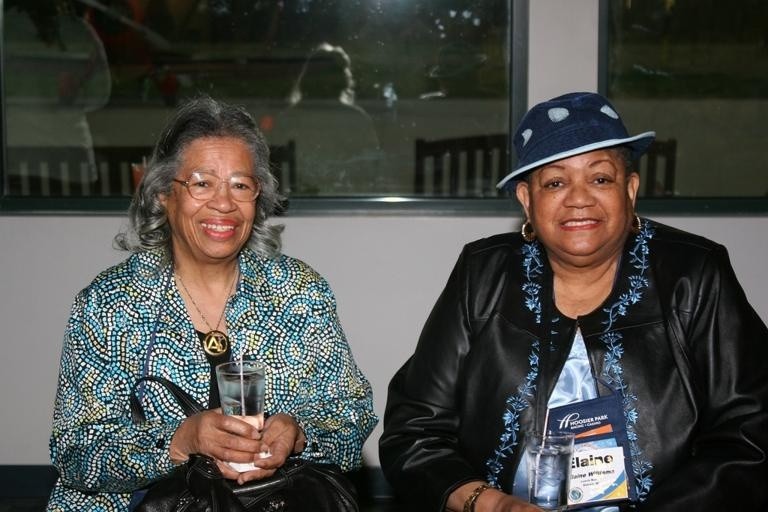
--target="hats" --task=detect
[492,90,656,194]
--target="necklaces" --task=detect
[171,260,239,357]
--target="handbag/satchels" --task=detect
[120,363,368,512]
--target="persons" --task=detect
[2,1,111,198]
[381,89,768,512]
[45,88,379,512]
[275,44,383,192]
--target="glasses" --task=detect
[164,169,263,203]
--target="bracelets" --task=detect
[462,483,492,512]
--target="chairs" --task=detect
[411,134,677,199]
[6,124,297,197]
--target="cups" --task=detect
[524,430,574,511]
[215,361,265,442]
